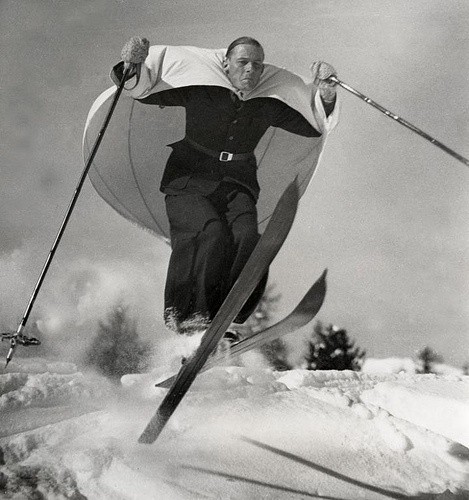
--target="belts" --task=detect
[185,137,254,162]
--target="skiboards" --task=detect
[135,171,327,442]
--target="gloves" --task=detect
[121,36,149,64]
[311,61,338,100]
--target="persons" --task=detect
[120,36,339,366]
